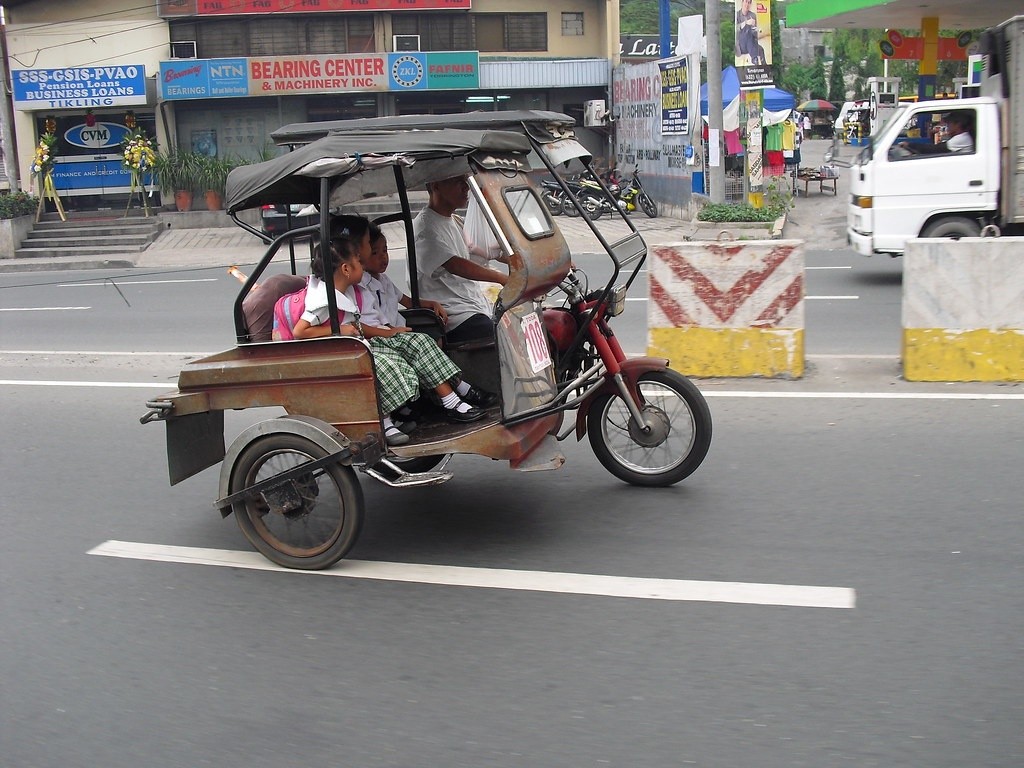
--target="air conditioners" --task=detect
[393,35,421,53]
[173,41,197,59]
[584,100,606,127]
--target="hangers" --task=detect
[785,119,790,122]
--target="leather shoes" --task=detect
[455,384,499,408]
[441,400,489,423]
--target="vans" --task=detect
[835,101,912,144]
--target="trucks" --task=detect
[847,14,1023,257]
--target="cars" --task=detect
[842,99,870,135]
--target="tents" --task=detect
[699,64,795,198]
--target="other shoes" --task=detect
[385,425,409,445]
[391,400,435,424]
[391,418,417,434]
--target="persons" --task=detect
[737,0,767,65]
[404,174,509,336]
[898,111,975,154]
[796,112,812,139]
[291,216,498,446]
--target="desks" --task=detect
[791,174,839,197]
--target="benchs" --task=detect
[241,274,447,377]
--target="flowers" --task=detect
[120,127,161,176]
[29,131,58,178]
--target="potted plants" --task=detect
[0,190,39,258]
[151,146,230,212]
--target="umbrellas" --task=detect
[796,98,838,137]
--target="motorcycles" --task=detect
[540,160,599,216]
[139,108,720,568]
[576,163,658,220]
[558,162,620,218]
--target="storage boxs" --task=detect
[820,166,839,178]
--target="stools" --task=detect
[452,338,505,403]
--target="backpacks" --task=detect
[270,279,363,342]
